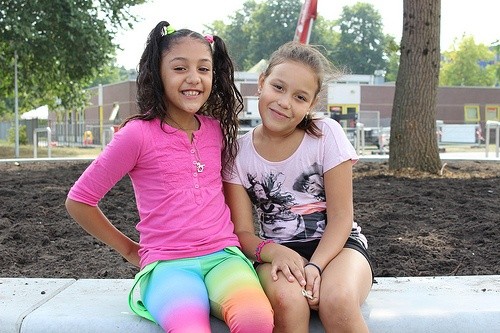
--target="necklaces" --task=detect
[169,115,206,173]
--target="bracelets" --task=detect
[255,238,277,265]
[302,262,323,277]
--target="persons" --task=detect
[216,40,378,333]
[63,19,277,333]
